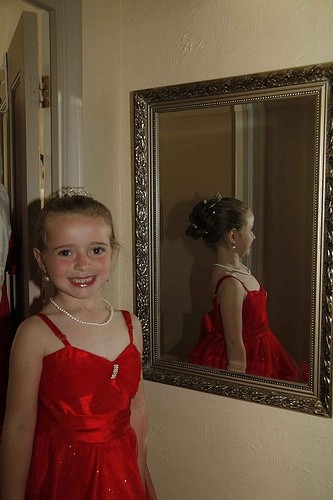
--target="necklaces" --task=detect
[213,263,251,275]
[49,297,114,326]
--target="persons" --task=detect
[0,187,157,500]
[186,191,300,383]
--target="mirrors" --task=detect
[129,60,333,418]
